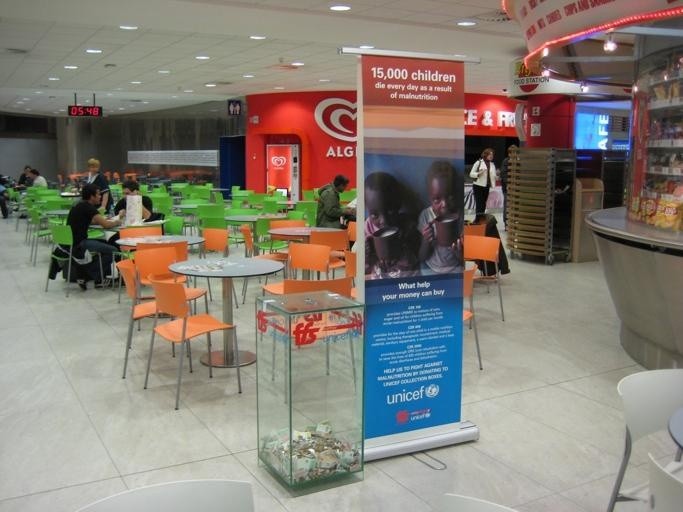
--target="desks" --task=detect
[666,404,683,456]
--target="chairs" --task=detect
[438,490,514,511]
[648,451,682,511]
[607,364,683,512]
[65,477,256,512]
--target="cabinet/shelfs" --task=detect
[505,143,571,259]
[628,53,683,205]
[578,178,600,262]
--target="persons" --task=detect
[0,157,50,223]
[113,179,152,220]
[417,160,463,268]
[468,147,496,225]
[65,183,124,291]
[498,143,520,232]
[84,158,114,211]
[315,173,356,229]
[339,194,357,228]
[363,171,418,275]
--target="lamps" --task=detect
[536,45,590,94]
[599,24,618,53]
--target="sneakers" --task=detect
[77,279,87,290]
[95,278,110,287]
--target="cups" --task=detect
[365,228,398,261]
[429,215,458,248]
